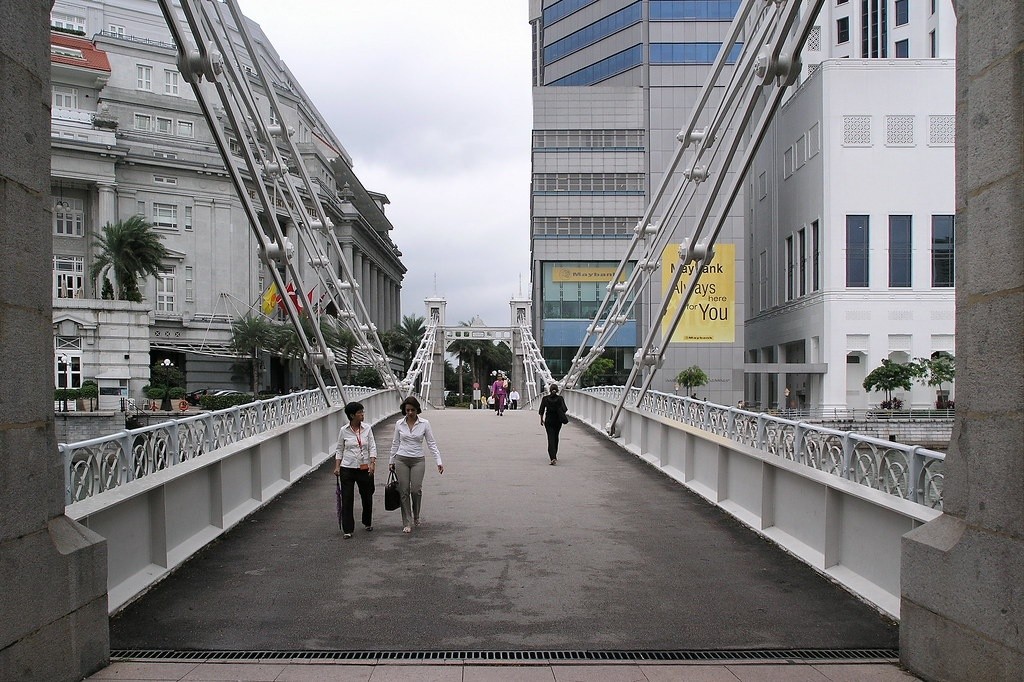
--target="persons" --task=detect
[538,383,568,464]
[288,386,293,394]
[333,401,377,540]
[388,396,444,533]
[480,387,519,410]
[492,373,509,416]
[693,393,697,399]
[704,398,706,401]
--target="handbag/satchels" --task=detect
[503,380,508,388]
[385,470,402,511]
[561,412,569,424]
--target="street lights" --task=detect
[476,347,484,409]
[161,359,174,411]
[57,353,72,412]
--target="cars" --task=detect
[185,388,252,408]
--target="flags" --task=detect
[262,282,338,321]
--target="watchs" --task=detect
[371,462,375,465]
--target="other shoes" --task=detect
[403,525,411,533]
[414,518,420,526]
[343,533,352,538]
[549,459,556,465]
[365,524,372,531]
[497,413,499,415]
[500,413,503,416]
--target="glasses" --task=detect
[497,376,502,377]
